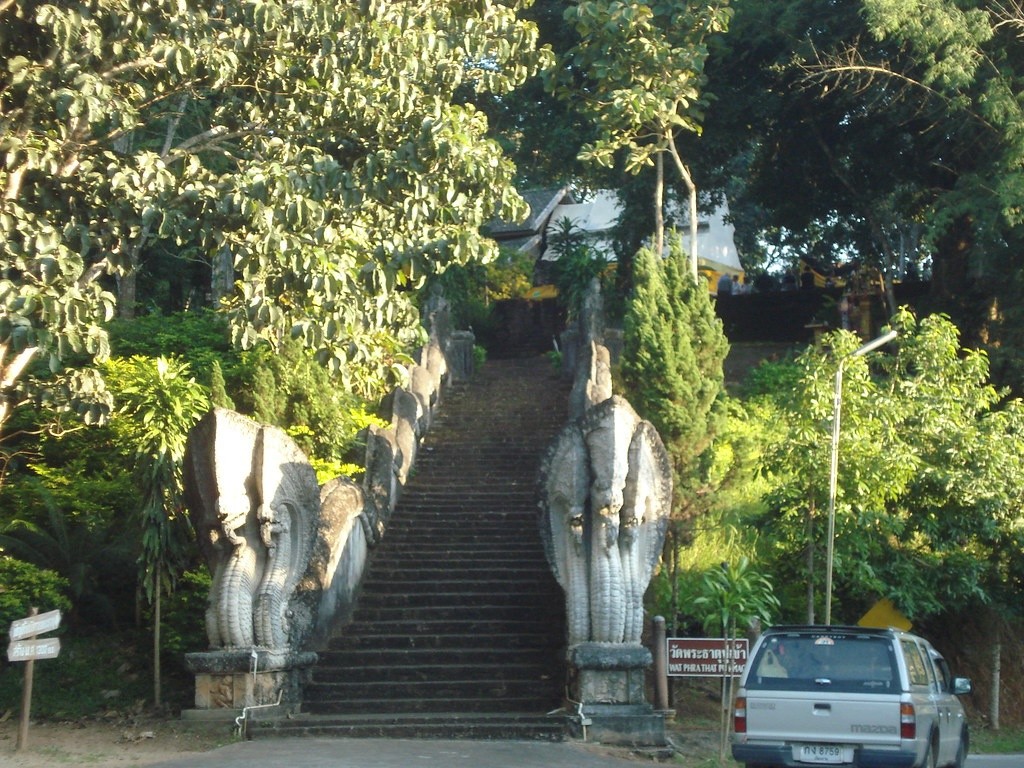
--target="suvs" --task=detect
[731,624,971,768]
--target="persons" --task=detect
[717,270,753,296]
[800,265,815,289]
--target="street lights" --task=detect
[825,330,896,626]
